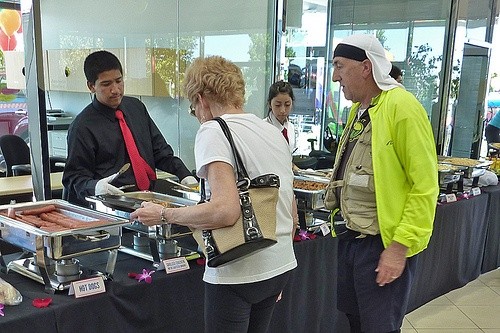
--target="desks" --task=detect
[0,183,500,333]
[0,171,180,196]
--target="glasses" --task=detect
[190,92,204,116]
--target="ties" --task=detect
[115,110,156,192]
[282,128,289,145]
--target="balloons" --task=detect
[0,8,23,52]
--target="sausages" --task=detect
[0,204,112,232]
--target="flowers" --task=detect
[32,298,51,307]
[294,229,317,241]
[0,303,5,318]
[127,268,155,286]
[460,192,473,200]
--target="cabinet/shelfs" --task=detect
[2,48,187,98]
[48,130,68,158]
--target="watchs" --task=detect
[160,207,168,224]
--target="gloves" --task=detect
[181,176,198,186]
[94,173,124,196]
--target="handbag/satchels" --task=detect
[193,117,280,267]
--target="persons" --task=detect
[262,81,296,152]
[388,65,403,86]
[485,109,500,158]
[129,56,297,332]
[323,34,440,333]
[62,51,197,206]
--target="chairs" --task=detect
[0,135,66,177]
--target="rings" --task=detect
[390,276,397,278]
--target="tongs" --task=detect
[109,163,136,190]
